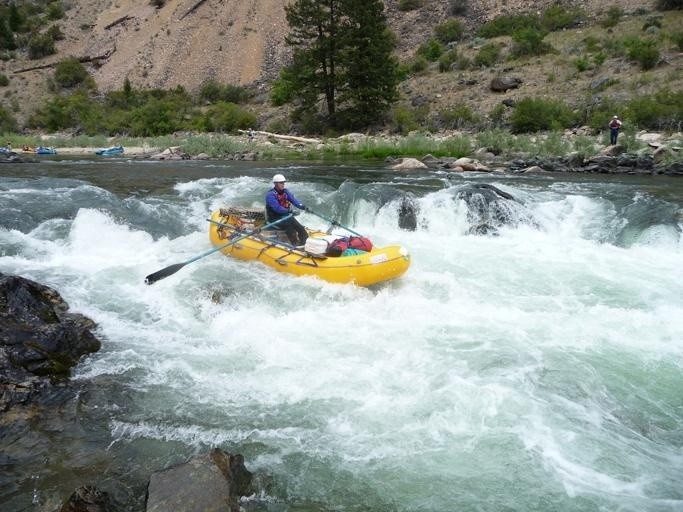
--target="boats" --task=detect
[95,146,124,155]
[20,147,56,155]
[209,208,409,286]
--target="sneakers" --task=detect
[291,239,306,246]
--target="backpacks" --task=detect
[326,235,372,256]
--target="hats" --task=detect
[273,174,286,183]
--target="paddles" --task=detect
[206,219,328,261]
[146,212,294,286]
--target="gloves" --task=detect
[305,207,313,213]
[289,209,301,215]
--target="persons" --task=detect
[264,172,308,250]
[247,127,252,145]
[608,113,622,147]
[21,144,34,151]
[5,138,12,152]
